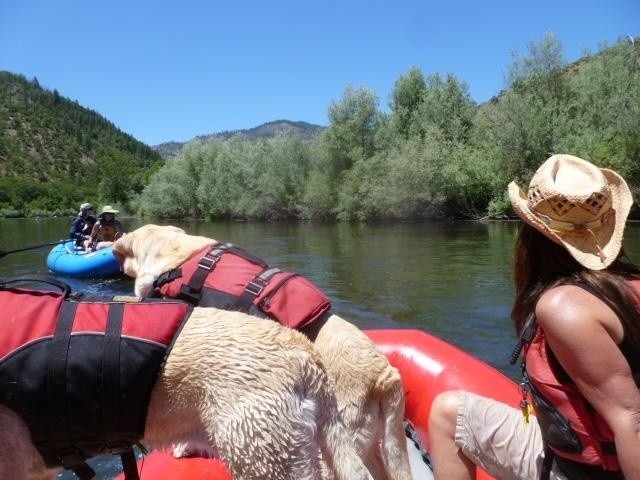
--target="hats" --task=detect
[98,206,120,218]
[78,203,93,216]
[508,154,634,270]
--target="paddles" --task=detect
[0,239,73,257]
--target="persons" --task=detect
[86,205,123,254]
[428,153,640,480]
[69,202,96,253]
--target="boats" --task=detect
[46,238,123,279]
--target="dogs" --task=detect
[0,285,371,480]
[111,225,411,480]
